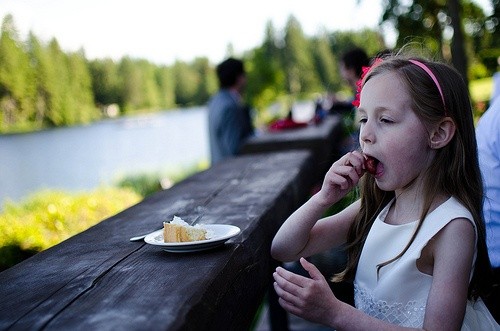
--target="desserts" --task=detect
[163,216,208,242]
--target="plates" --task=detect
[144,224,241,252]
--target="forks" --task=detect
[129,215,201,242]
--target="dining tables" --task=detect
[244,111,342,170]
[0,151,319,331]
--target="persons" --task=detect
[492,59,499,99]
[319,43,374,154]
[207,58,256,164]
[472,89,500,324]
[269,55,480,331]
